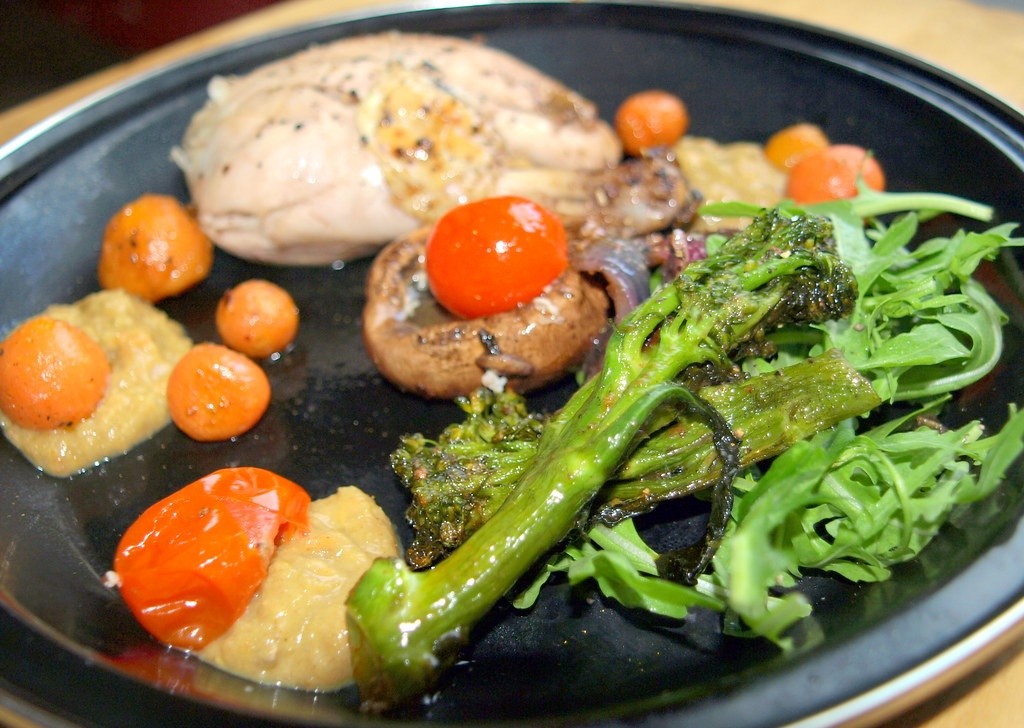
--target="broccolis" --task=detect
[343,206,853,723]
[392,353,881,571]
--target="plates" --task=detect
[0,0,1024,728]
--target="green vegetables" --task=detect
[509,189,1024,653]
